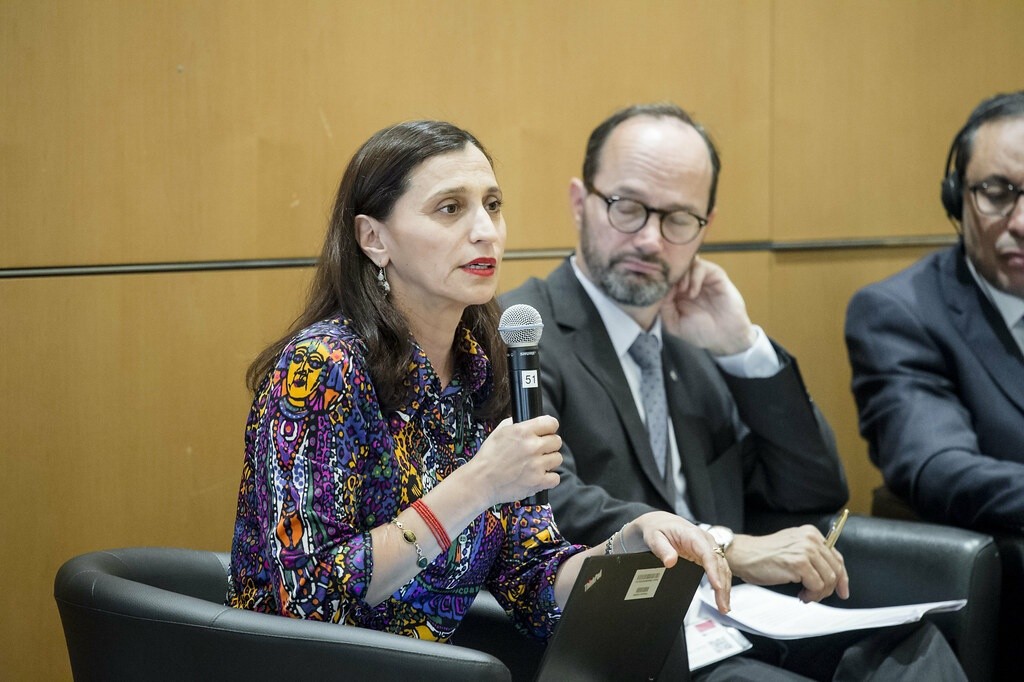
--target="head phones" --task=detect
[939,95,1024,222]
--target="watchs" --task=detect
[707,526,733,551]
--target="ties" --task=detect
[629,333,695,520]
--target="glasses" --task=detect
[584,183,709,245]
[965,176,1024,216]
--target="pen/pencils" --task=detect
[797,509,850,601]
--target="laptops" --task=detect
[511,548,706,682]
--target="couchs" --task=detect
[53,548,511,682]
[871,486,1024,682]
[823,514,1004,682]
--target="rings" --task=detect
[714,547,725,558]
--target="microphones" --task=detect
[497,304,550,507]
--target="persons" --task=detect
[496,102,967,681]
[230,121,732,643]
[845,89,1024,536]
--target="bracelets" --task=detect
[606,533,618,555]
[392,518,427,570]
[620,524,627,554]
[411,499,451,552]
